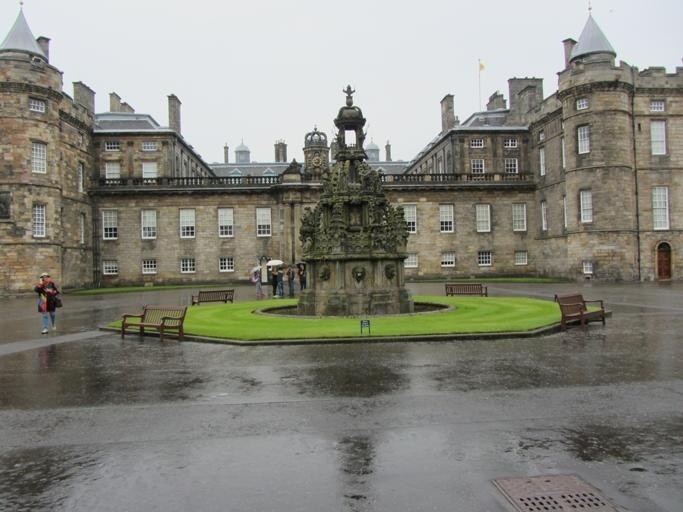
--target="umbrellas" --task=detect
[265,259,282,266]
[250,265,261,275]
[295,262,306,267]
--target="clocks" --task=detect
[312,157,322,167]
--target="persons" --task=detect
[251,271,263,298]
[272,266,306,298]
[32,270,59,335]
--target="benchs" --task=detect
[121,303,187,341]
[192,289,234,306]
[445,283,487,297]
[554,293,605,331]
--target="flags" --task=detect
[478,62,484,70]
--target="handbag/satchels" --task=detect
[54,298,62,307]
